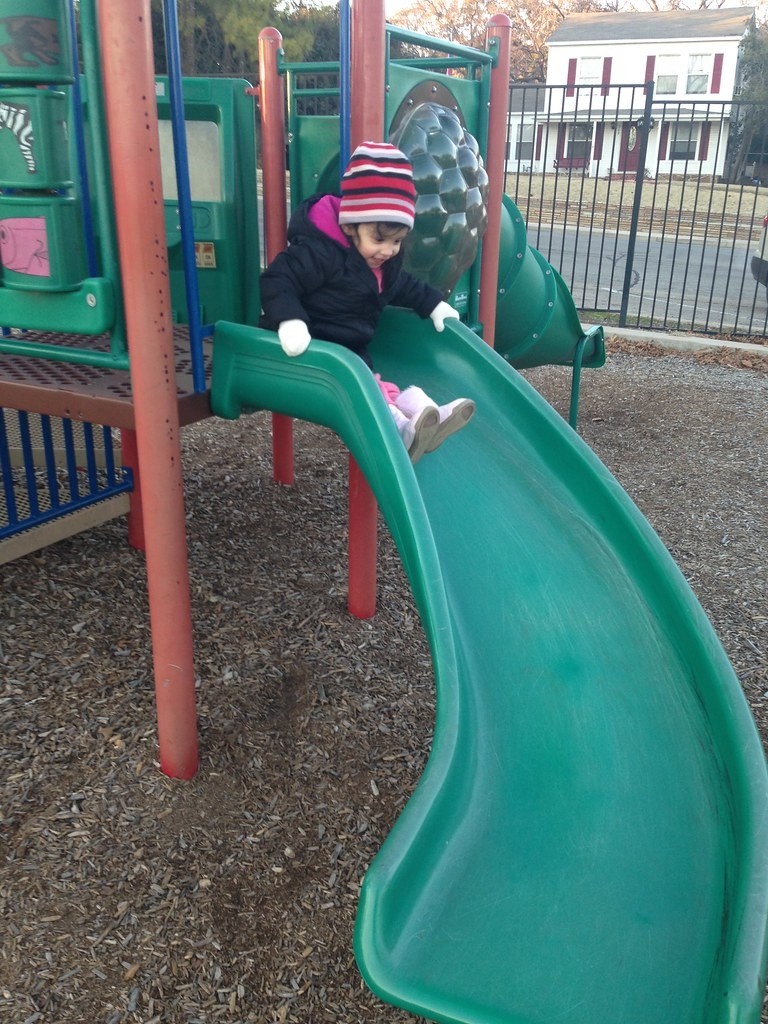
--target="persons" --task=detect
[259,140,476,465]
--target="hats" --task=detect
[339,141,418,230]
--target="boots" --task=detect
[386,403,440,465]
[395,385,476,453]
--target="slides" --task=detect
[207,294,768,1024]
[471,193,607,370]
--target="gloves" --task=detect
[430,301,460,333]
[278,320,312,358]
